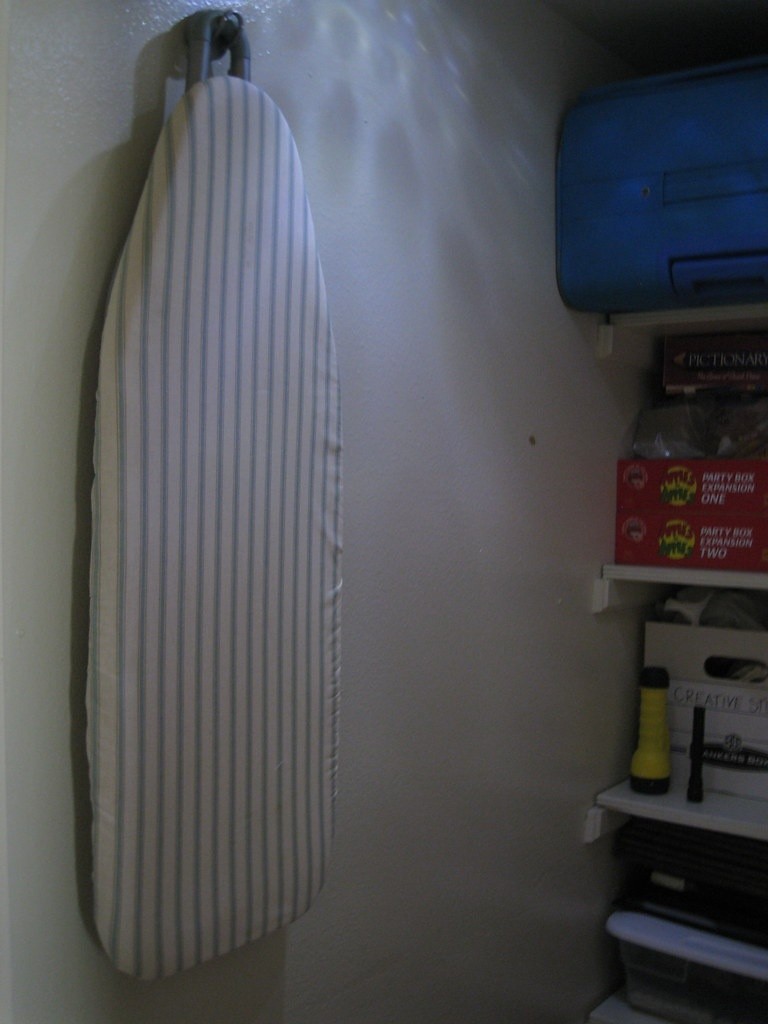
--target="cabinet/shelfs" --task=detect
[587,303,768,1024]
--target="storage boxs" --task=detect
[662,331,768,394]
[616,454,768,515]
[605,910,768,1024]
[615,514,768,574]
[641,621,768,804]
